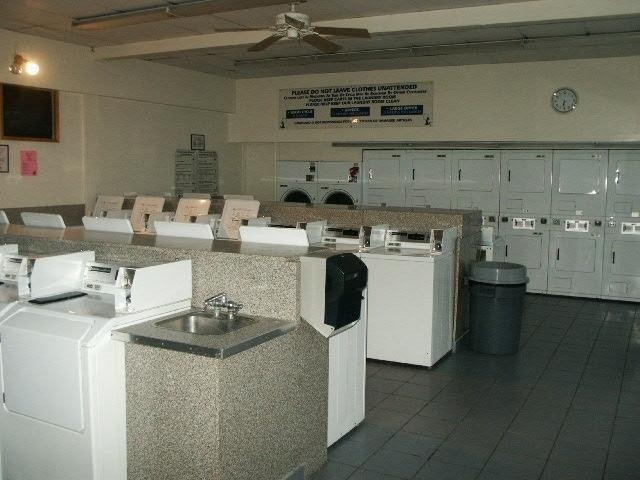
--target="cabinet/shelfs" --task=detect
[497,141,553,296]
[601,141,640,302]
[547,140,609,300]
[405,141,452,208]
[360,140,404,210]
[450,142,501,275]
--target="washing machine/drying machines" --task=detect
[0,159,507,479]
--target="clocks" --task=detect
[551,87,579,114]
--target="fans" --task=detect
[215,2,370,53]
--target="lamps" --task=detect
[9,55,39,75]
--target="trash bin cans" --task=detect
[468,261,529,355]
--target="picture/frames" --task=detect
[0,145,9,173]
[191,134,205,150]
